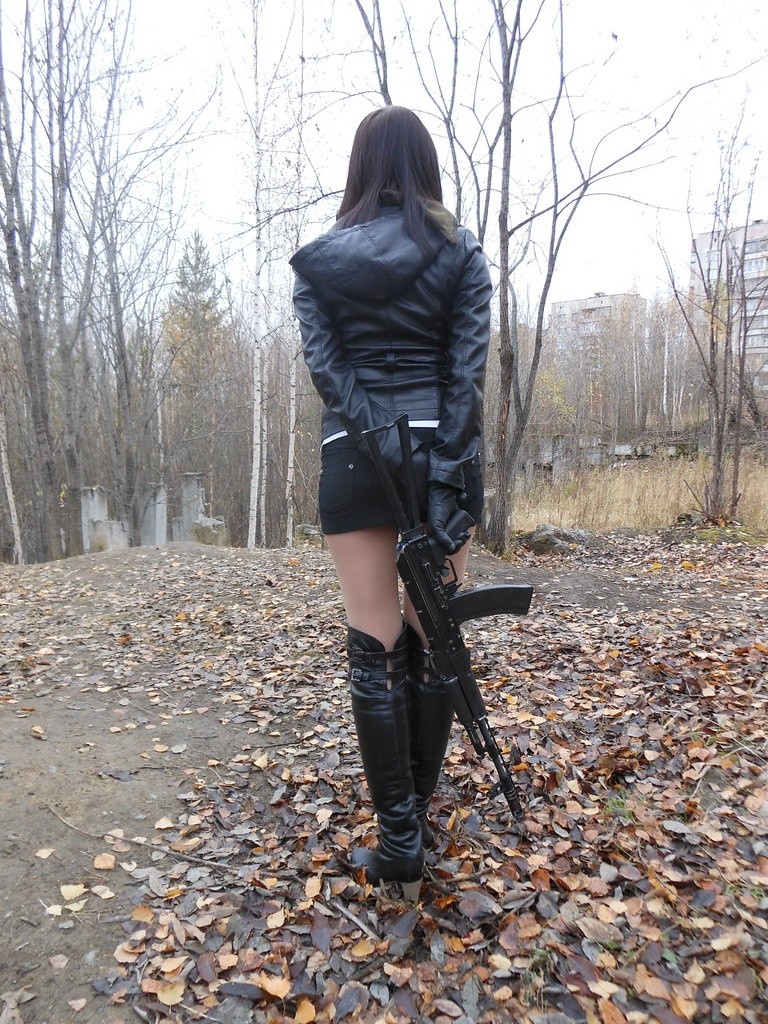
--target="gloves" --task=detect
[427,481,471,556]
[396,448,467,517]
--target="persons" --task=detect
[288,106,492,904]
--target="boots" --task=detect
[406,622,453,852]
[346,623,425,886]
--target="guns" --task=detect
[360,413,532,827]
